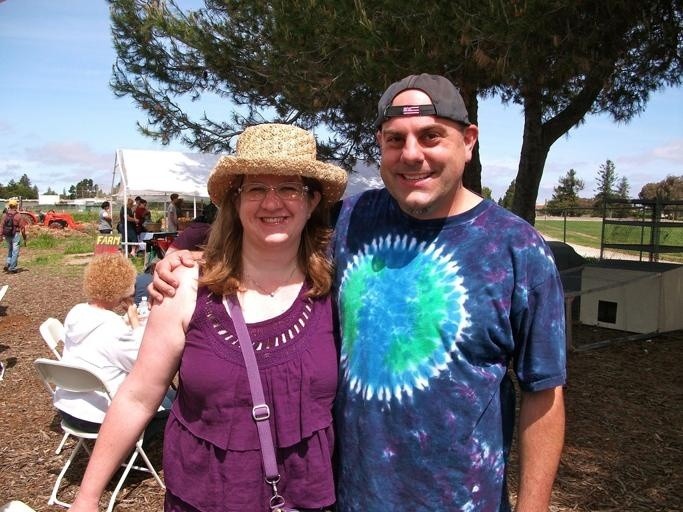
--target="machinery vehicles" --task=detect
[17,208,77,230]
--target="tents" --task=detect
[108,147,382,259]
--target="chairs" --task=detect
[33,359,167,511]
[39,317,66,361]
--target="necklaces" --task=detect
[242,262,298,297]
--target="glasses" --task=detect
[237,182,310,202]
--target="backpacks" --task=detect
[2,211,19,238]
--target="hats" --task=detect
[373,72,472,129]
[207,123,349,212]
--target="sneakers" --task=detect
[4,265,18,274]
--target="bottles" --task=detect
[137,296,151,330]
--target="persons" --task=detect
[148,73,567,511]
[68,126,348,511]
[0,200,27,274]
[96,193,218,258]
[54,250,178,434]
